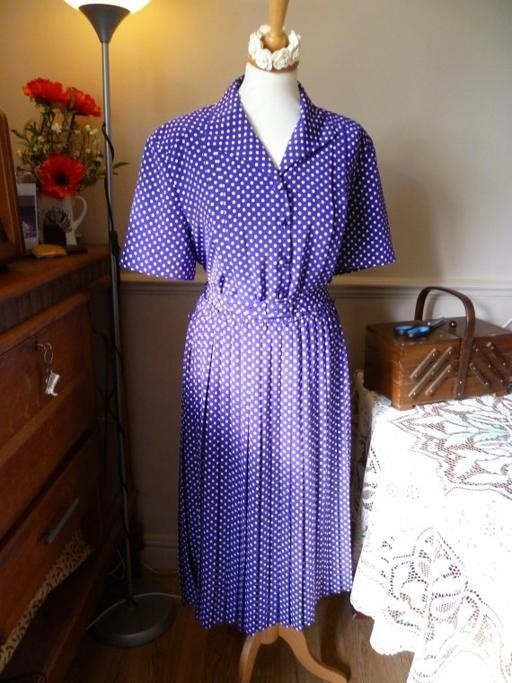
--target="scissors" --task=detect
[393,318,447,339]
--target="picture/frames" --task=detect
[15,182,39,256]
[1,110,26,266]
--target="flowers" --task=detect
[8,79,130,206]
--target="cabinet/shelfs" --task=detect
[0,248,114,683]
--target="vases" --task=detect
[38,194,89,246]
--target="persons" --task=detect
[120,21,395,637]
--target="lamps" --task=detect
[59,0,175,648]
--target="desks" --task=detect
[345,368,512,683]
